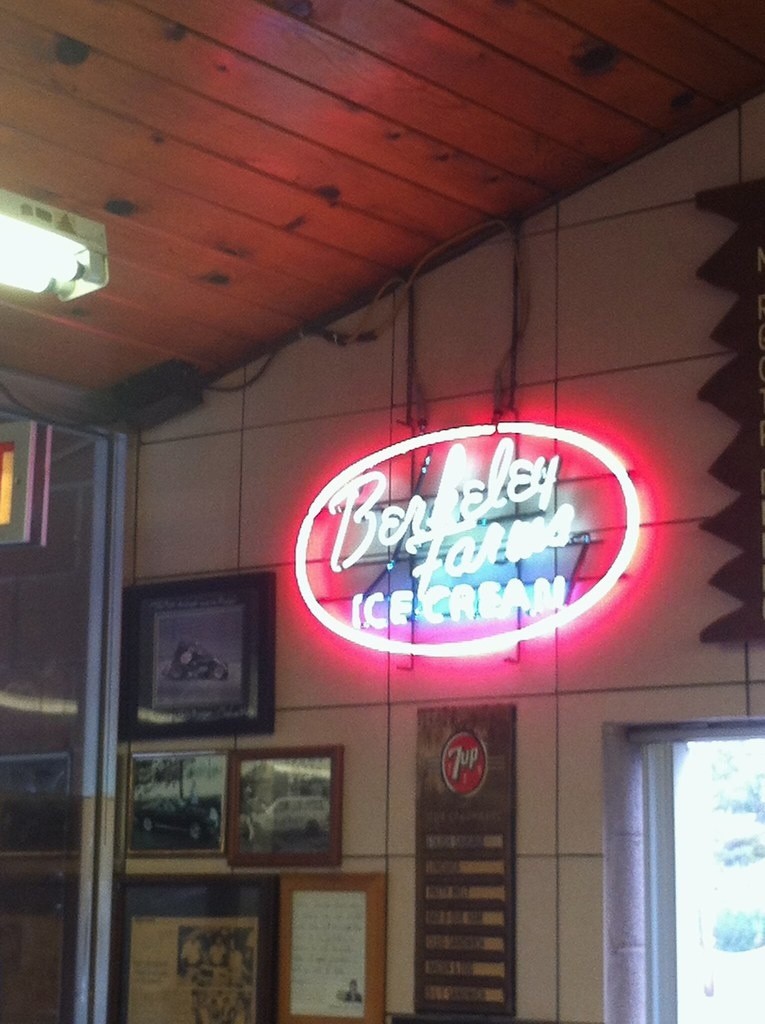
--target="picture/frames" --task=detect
[116,572,276,743]
[119,738,226,860]
[227,743,348,870]
[106,873,278,1024]
[277,870,391,1024]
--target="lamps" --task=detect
[0,186,112,305]
[110,359,205,433]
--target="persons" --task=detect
[191,989,250,1024]
[346,979,361,1002]
[182,930,245,983]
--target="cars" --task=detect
[132,793,220,842]
[162,634,231,681]
[242,792,330,838]
